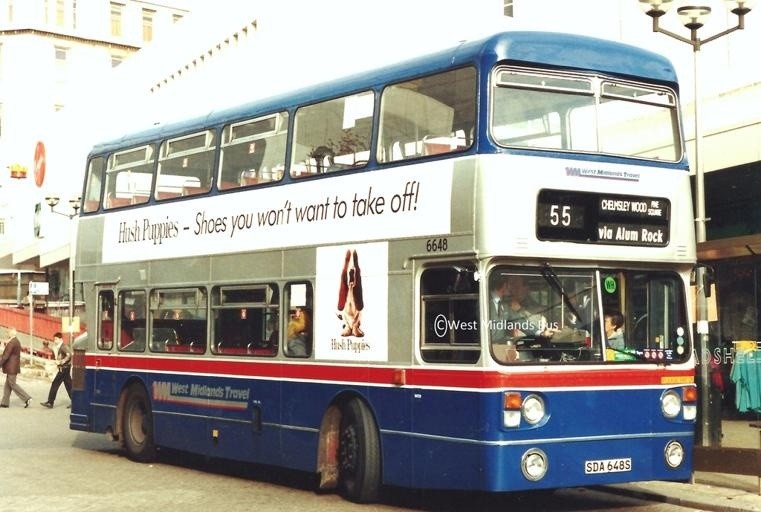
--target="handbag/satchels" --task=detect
[58,354,71,368]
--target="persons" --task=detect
[40,332,72,409]
[473,275,625,350]
[23,291,36,311]
[0,327,32,408]
[42,341,54,359]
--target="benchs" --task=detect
[99,317,274,355]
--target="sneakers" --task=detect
[40,402,53,408]
[67,402,72,409]
[0,403,9,408]
[23,397,33,408]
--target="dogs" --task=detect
[336,249,365,337]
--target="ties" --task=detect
[497,301,502,317]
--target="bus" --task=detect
[66,34,703,505]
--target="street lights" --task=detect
[45,194,79,376]
[638,0,722,446]
